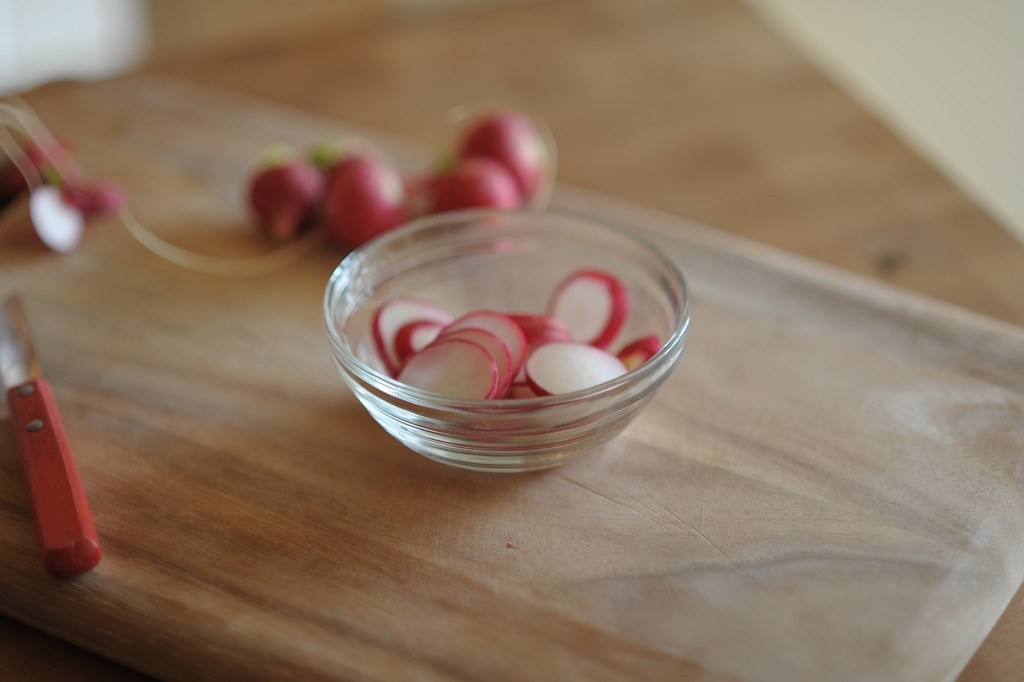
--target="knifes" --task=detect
[0,292,103,581]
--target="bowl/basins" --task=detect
[320,206,692,473]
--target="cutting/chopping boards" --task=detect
[0,69,1024,682]
